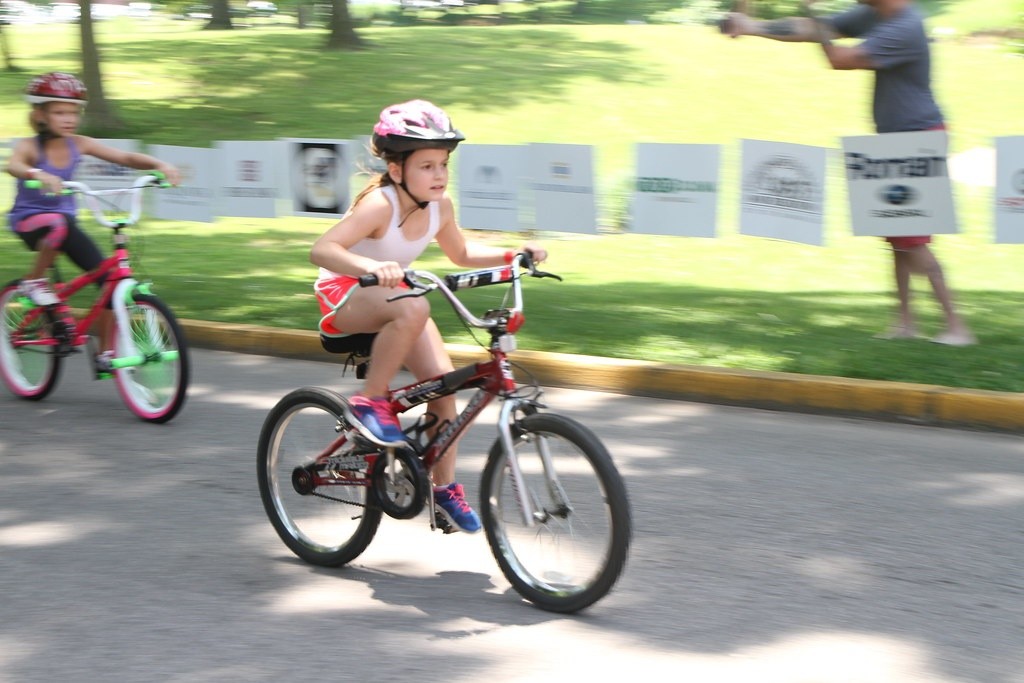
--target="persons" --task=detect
[309,98,547,534]
[717,1,976,348]
[6,72,180,373]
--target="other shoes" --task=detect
[873,323,980,348]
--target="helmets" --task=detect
[372,98,466,155]
[24,72,89,106]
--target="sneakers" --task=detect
[343,389,408,449]
[94,348,136,376]
[431,481,481,535]
[19,276,60,308]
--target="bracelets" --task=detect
[504,249,512,263]
[25,167,43,179]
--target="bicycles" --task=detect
[0,171,192,423]
[257,251,633,617]
